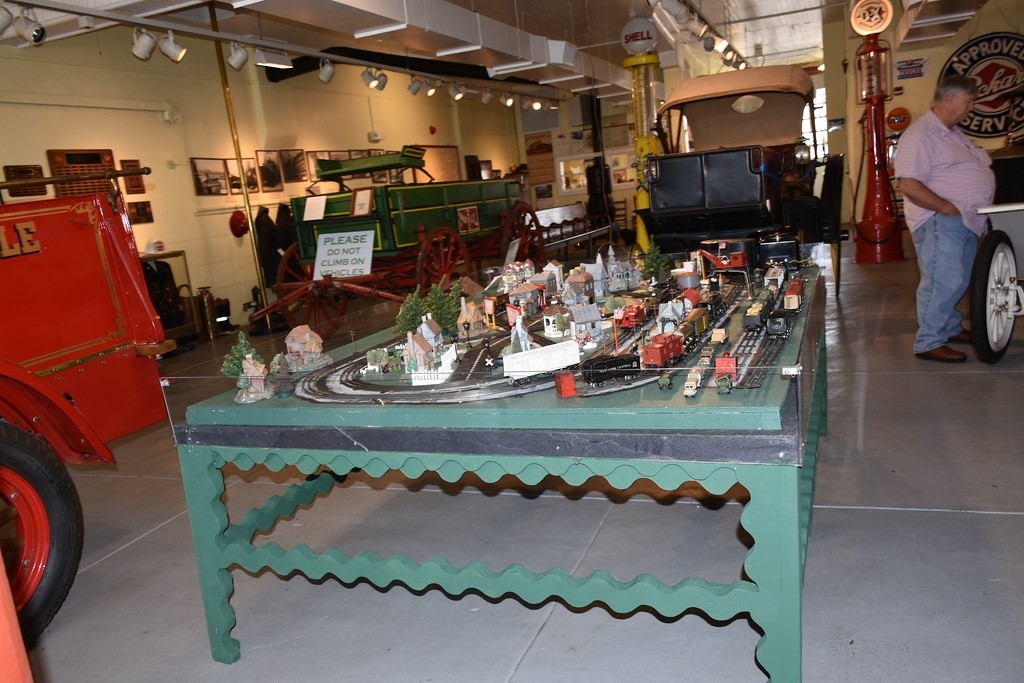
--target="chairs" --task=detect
[612,199,627,230]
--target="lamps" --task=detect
[374,69,387,90]
[406,75,421,95]
[131,27,157,60]
[353,0,407,39]
[318,59,336,83]
[421,78,435,96]
[157,29,186,62]
[361,67,380,88]
[448,83,463,100]
[687,20,746,71]
[253,49,293,68]
[480,88,493,104]
[232,0,263,8]
[226,42,248,70]
[0,0,48,46]
[491,37,633,111]
[436,14,482,56]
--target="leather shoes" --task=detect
[915,344,967,364]
[947,330,973,344]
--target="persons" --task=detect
[894,75,994,362]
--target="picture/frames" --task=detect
[190,149,401,196]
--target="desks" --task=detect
[156,263,828,683]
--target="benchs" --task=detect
[515,201,612,263]
